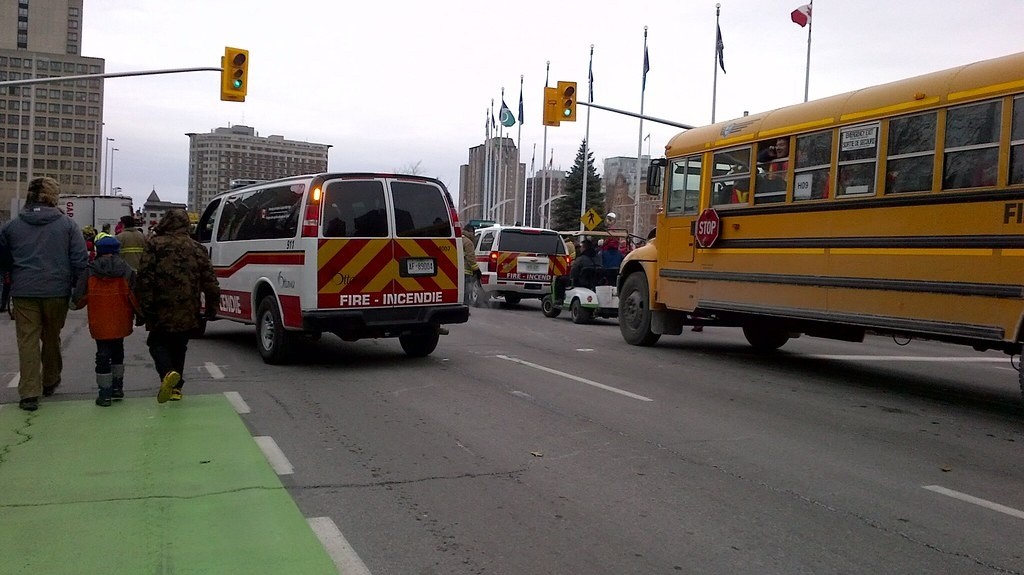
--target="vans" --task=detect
[189,172,469,364]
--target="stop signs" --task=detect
[695,208,719,248]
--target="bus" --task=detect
[604,50,1024,398]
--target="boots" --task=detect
[111,365,125,399]
[95,373,112,406]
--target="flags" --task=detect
[643,46,649,91]
[486,70,552,173]
[717,23,726,74]
[588,61,594,103]
[791,5,812,28]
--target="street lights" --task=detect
[104,138,114,195]
[110,148,118,195]
[113,187,121,196]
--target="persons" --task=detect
[0,176,221,413]
[727,136,1024,202]
[554,213,645,306]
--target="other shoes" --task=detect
[19,396,38,410]
[43,379,61,396]
[156,371,183,403]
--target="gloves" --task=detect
[472,268,482,280]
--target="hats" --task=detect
[23,177,65,215]
[95,237,121,256]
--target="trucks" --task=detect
[57,194,144,240]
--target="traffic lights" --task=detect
[223,47,249,95]
[557,81,576,121]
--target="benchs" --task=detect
[576,267,619,290]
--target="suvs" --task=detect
[469,225,571,308]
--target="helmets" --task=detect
[605,213,616,228]
